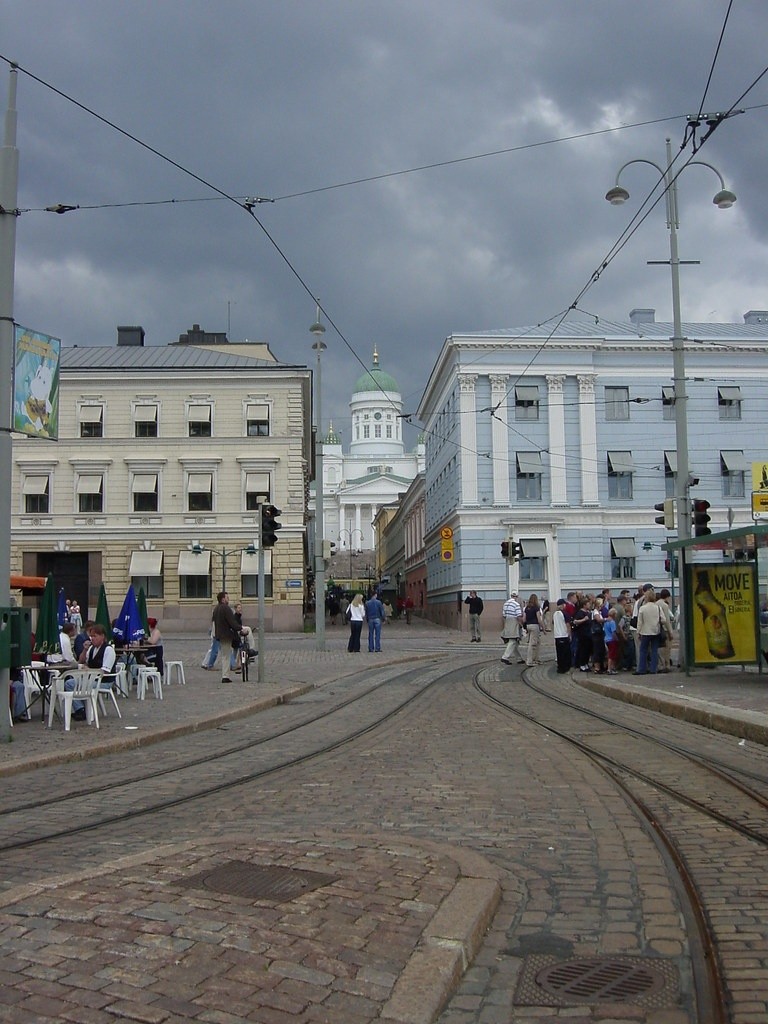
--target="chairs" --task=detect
[115,661,185,700]
[8,665,122,731]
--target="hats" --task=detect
[557,599,566,605]
[511,591,519,596]
[661,588,670,598]
[621,589,630,594]
[642,584,653,592]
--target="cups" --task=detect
[78,664,83,670]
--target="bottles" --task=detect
[694,571,735,659]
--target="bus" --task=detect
[324,579,376,599]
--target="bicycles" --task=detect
[235,627,259,682]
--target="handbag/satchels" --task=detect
[345,603,352,621]
[659,624,667,648]
[569,616,587,630]
[590,610,603,634]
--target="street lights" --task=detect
[192,545,256,592]
[641,542,675,616]
[310,296,345,652]
[395,571,402,596]
[378,569,383,582]
[337,519,364,579]
[605,139,738,674]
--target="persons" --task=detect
[346,595,365,653]
[10,667,28,723]
[465,590,484,643]
[201,592,259,683]
[59,600,163,721]
[329,595,414,625]
[365,591,386,652]
[501,584,682,675]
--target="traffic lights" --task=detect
[500,542,509,557]
[509,541,521,557]
[694,498,711,537]
[654,499,674,529]
[322,540,337,559]
[259,504,282,549]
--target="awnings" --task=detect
[77,475,102,494]
[187,474,211,494]
[178,550,211,576]
[128,550,164,577]
[246,473,270,493]
[516,384,747,560]
[79,405,270,423]
[131,474,158,493]
[23,475,48,496]
[241,550,273,575]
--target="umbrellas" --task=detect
[34,574,62,654]
[95,583,150,650]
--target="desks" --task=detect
[16,666,79,725]
[114,645,156,675]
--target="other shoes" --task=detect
[349,650,360,652]
[556,662,672,675]
[517,660,525,664]
[222,678,230,683]
[375,650,382,652]
[501,658,512,665]
[19,714,28,722]
[71,708,86,720]
[201,665,212,671]
[527,663,538,666]
[470,638,481,642]
